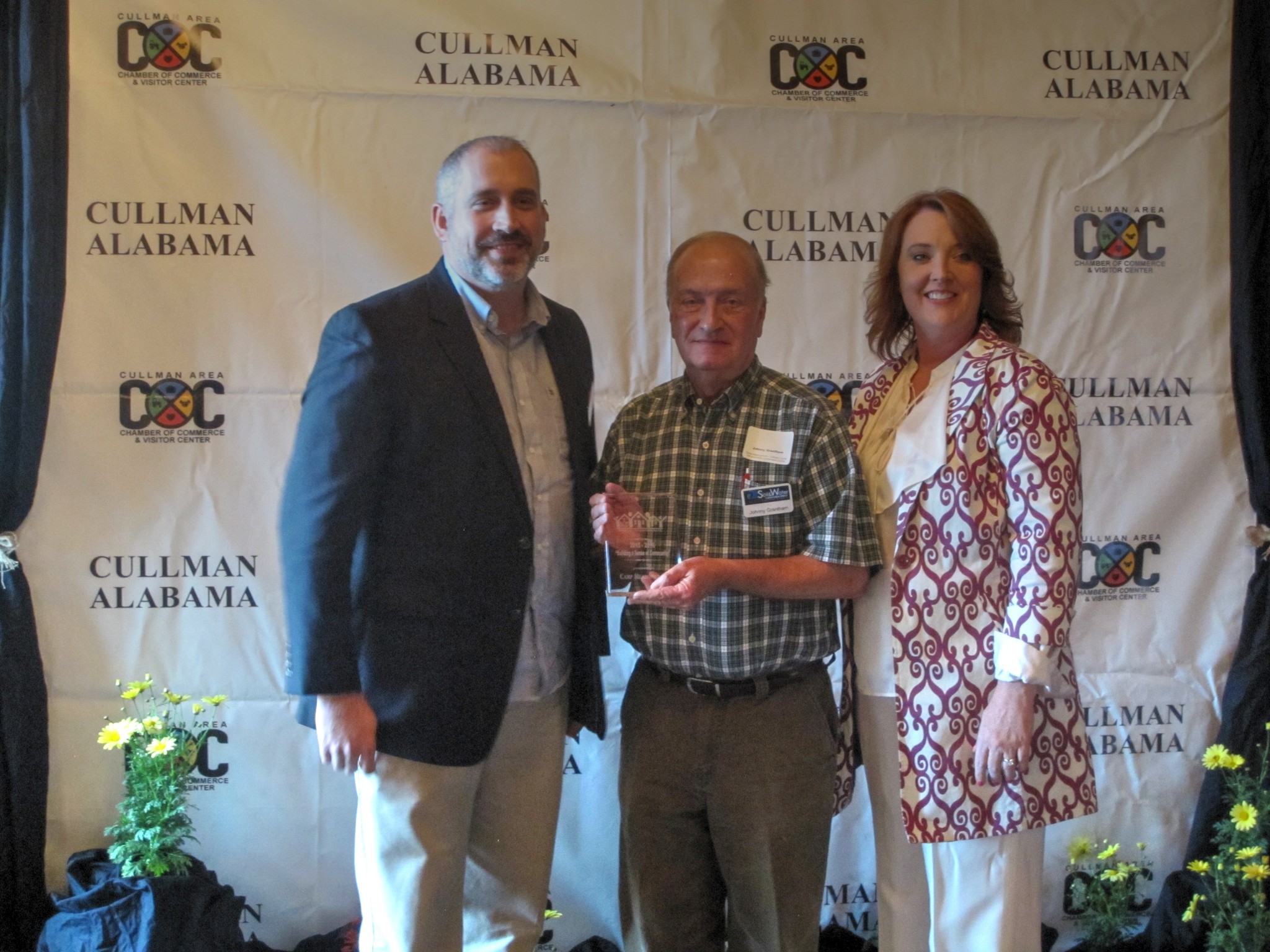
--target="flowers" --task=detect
[1053,711,1270,952]
[91,670,230,874]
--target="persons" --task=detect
[275,136,619,950]
[836,187,1102,951]
[580,232,886,950]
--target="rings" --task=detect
[1004,758,1016,766]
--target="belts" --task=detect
[641,656,823,699]
